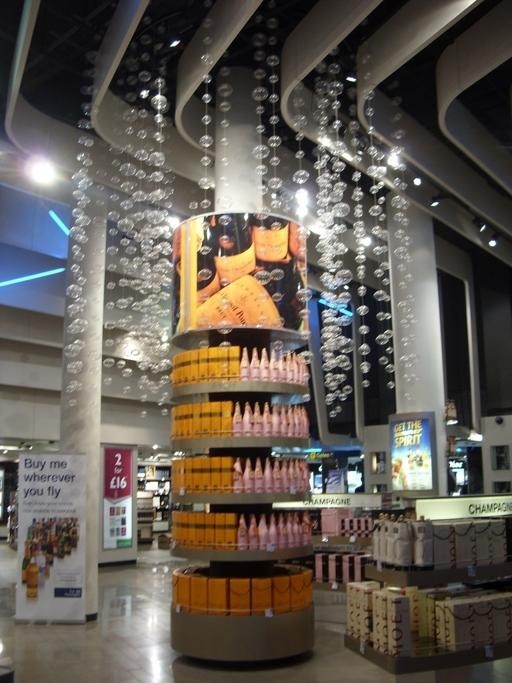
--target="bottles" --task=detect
[22,517,79,599]
[408,445,429,470]
[197,213,306,332]
[233,346,312,550]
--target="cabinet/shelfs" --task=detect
[343,562,511,682]
[169,211,316,664]
[138,461,172,543]
